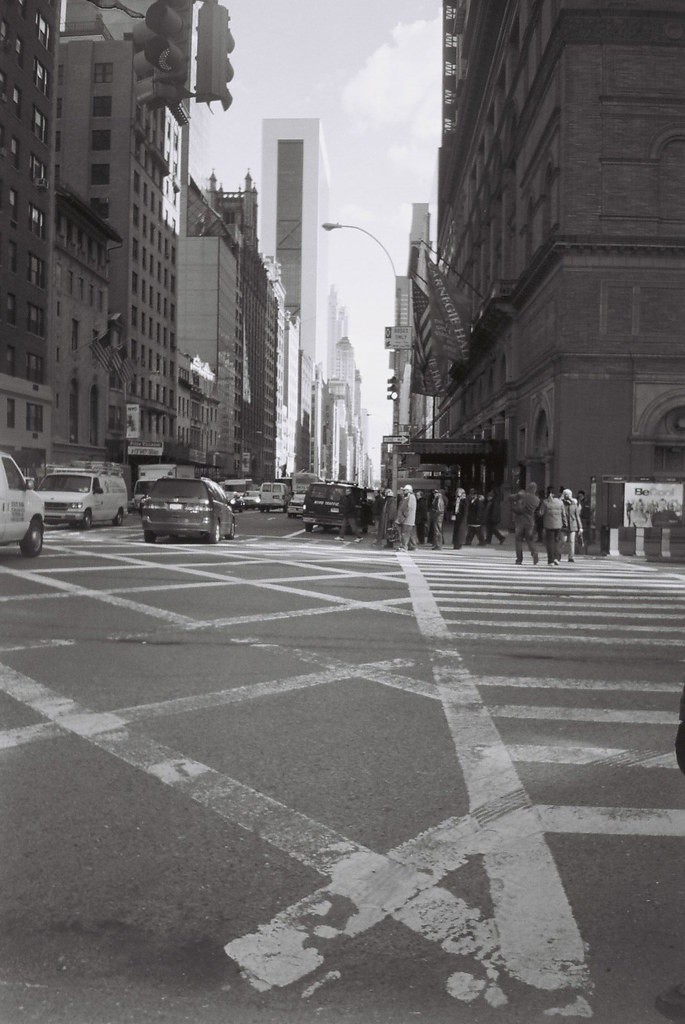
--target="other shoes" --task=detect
[396,547,406,552]
[500,537,505,544]
[554,559,559,565]
[334,536,344,541]
[478,543,486,546]
[354,537,363,542]
[515,562,521,565]
[569,559,574,562]
[485,540,491,544]
[549,562,555,566]
[432,546,441,550]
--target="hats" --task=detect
[401,485,413,490]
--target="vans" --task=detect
[34,460,129,530]
[223,478,253,513]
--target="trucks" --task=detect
[130,463,197,518]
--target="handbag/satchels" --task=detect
[511,492,525,513]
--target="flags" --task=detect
[89,338,135,384]
[410,279,449,397]
[425,250,474,372]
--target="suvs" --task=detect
[301,480,369,535]
[258,482,289,514]
[140,476,236,544]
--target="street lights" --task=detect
[322,221,404,524]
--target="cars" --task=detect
[242,490,260,511]
[1,449,47,559]
[222,491,246,513]
[285,493,306,519]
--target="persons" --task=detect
[535,486,590,565]
[371,485,506,552]
[334,488,363,543]
[626,497,682,527]
[511,482,541,565]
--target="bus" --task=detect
[291,472,317,493]
